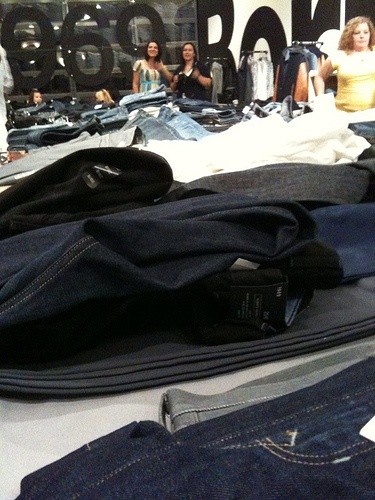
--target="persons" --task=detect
[132,41,172,94]
[170,42,211,99]
[0,43,117,165]
[313,16,375,112]
